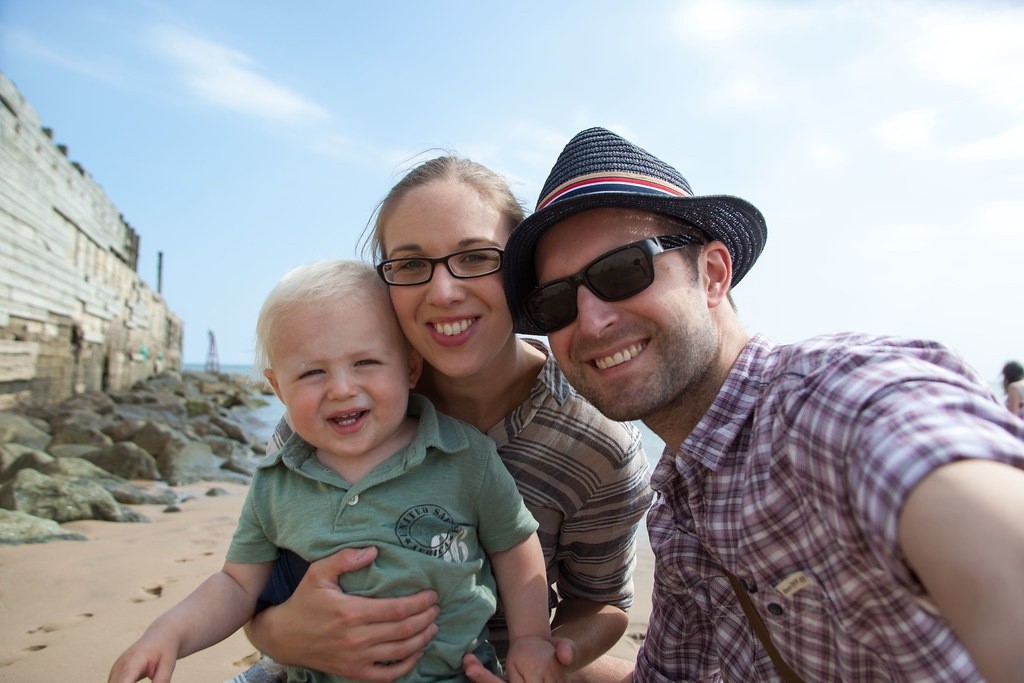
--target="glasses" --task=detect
[376,247,504,286]
[520,233,704,334]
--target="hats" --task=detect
[499,127,768,335]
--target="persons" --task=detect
[464,128,1023,683]
[106,147,655,682]
